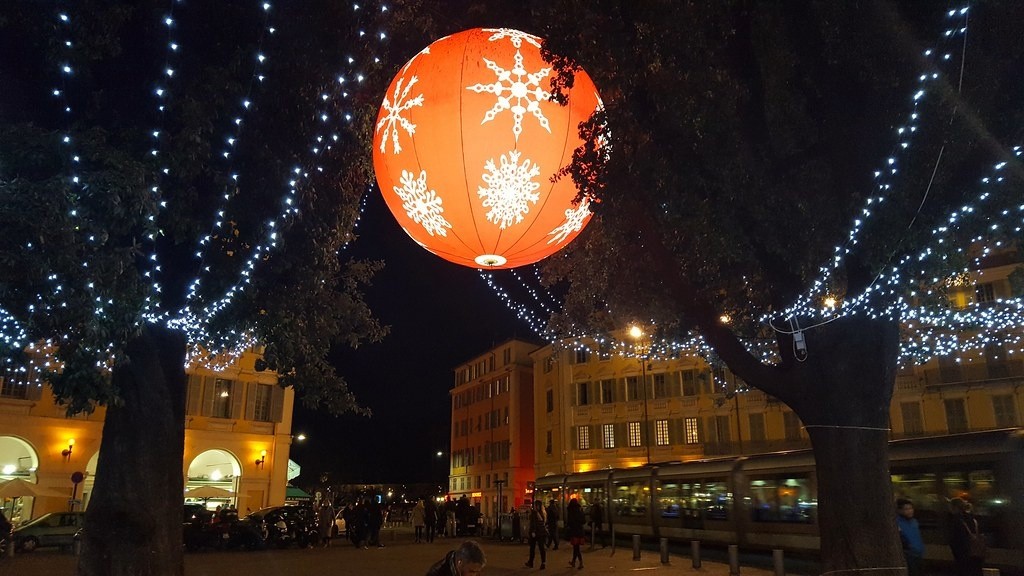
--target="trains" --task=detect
[534,427,1023,574]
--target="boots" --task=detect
[578,560,584,569]
[568,559,575,567]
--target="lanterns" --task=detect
[370,25,614,271]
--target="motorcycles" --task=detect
[185,506,322,550]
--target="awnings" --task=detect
[286,486,313,499]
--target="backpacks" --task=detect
[961,517,988,558]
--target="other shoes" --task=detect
[540,563,546,570]
[553,545,559,550]
[545,543,550,548]
[525,561,533,568]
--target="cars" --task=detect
[242,505,313,524]
[315,506,349,537]
[12,511,85,552]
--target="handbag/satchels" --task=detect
[571,536,586,545]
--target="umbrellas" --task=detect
[0,477,75,521]
[182,485,250,510]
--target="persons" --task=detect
[426,540,487,576]
[216,505,222,516]
[895,498,926,576]
[944,497,987,576]
[223,504,232,517]
[293,486,606,569]
[229,505,238,518]
